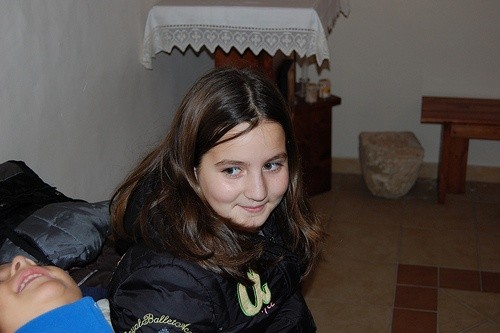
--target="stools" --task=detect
[420,95,500,207]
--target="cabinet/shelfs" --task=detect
[292,82,343,197]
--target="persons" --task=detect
[0,255,115,333]
[108,66,327,333]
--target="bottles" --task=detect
[319,79,330,98]
[299,77,310,97]
[306,83,317,103]
